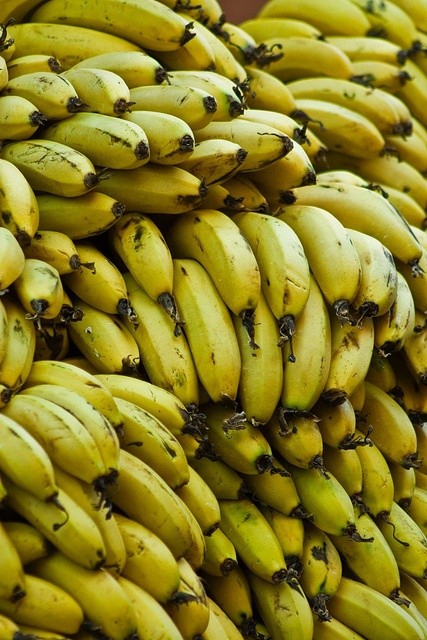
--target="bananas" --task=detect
[1,1,427,640]
